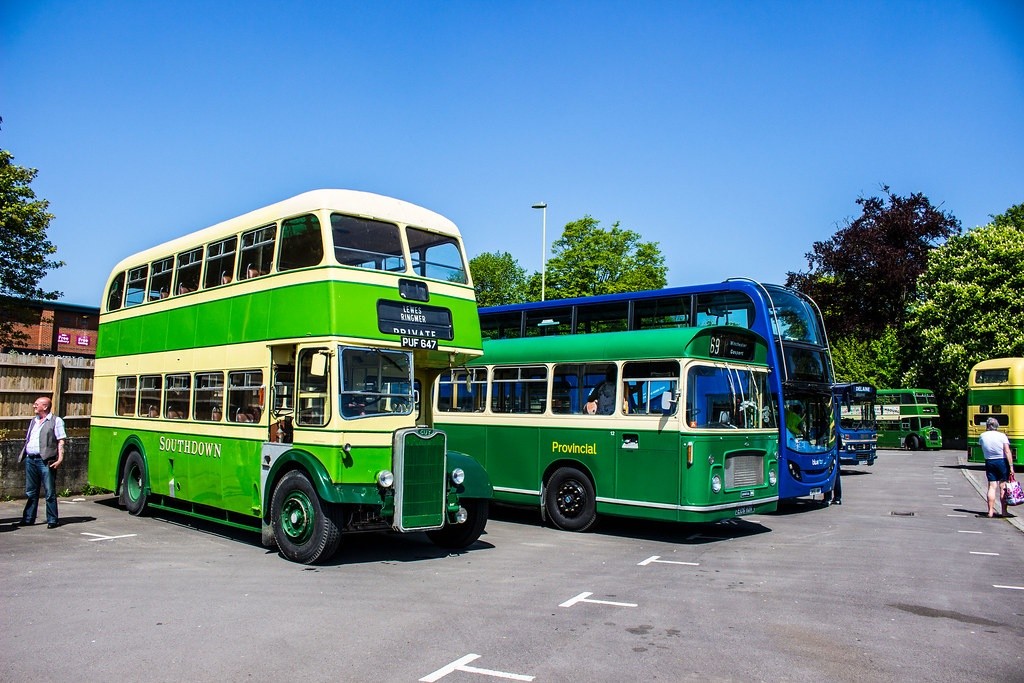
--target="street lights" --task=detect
[531,201,547,303]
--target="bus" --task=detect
[86,187,497,567]
[478,276,839,518]
[432,325,779,533]
[875,388,944,451]
[831,379,879,468]
[966,357,1024,474]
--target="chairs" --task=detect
[312,387,327,424]
[246,263,270,279]
[196,402,212,421]
[211,404,237,421]
[178,282,196,295]
[159,285,169,299]
[235,406,261,423]
[149,405,160,418]
[221,269,233,285]
[167,405,188,419]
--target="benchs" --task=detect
[510,408,545,414]
[633,408,663,414]
[479,406,513,413]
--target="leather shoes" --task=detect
[12,522,33,526]
[47,524,56,529]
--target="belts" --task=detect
[27,454,41,459]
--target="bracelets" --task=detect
[1009,464,1013,466]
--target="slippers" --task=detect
[1002,513,1013,518]
[986,513,995,518]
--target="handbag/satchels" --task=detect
[1003,475,1024,506]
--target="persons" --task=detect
[822,433,843,504]
[979,418,1014,517]
[19,397,67,528]
[588,365,629,415]
